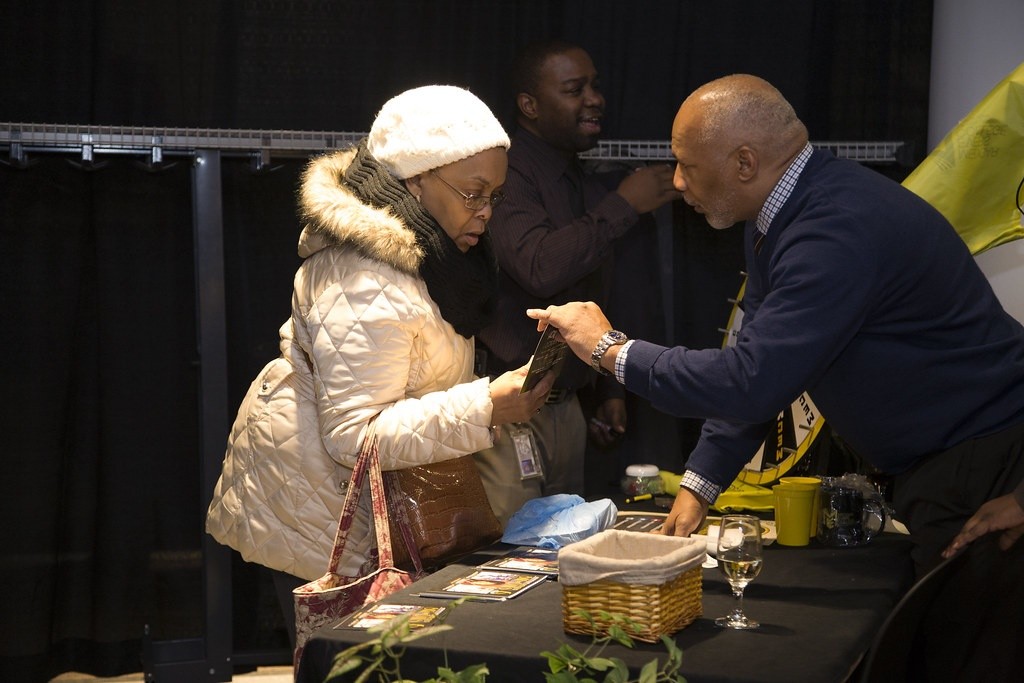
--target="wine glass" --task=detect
[713,514,765,630]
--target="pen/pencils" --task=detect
[409,592,507,604]
[625,493,657,504]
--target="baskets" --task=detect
[557,528,707,644]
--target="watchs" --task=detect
[591,331,628,376]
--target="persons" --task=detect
[205,42,677,652]
[525,74,1023,594]
[940,487,1024,557]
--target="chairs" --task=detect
[864,526,999,683]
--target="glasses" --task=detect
[430,168,508,211]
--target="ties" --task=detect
[752,226,766,256]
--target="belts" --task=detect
[544,389,575,404]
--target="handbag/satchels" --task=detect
[293,413,429,683]
[383,454,503,564]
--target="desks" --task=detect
[291,488,915,683]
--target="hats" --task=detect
[365,85,511,180]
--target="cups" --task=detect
[778,478,821,537]
[771,484,815,546]
[820,486,886,549]
